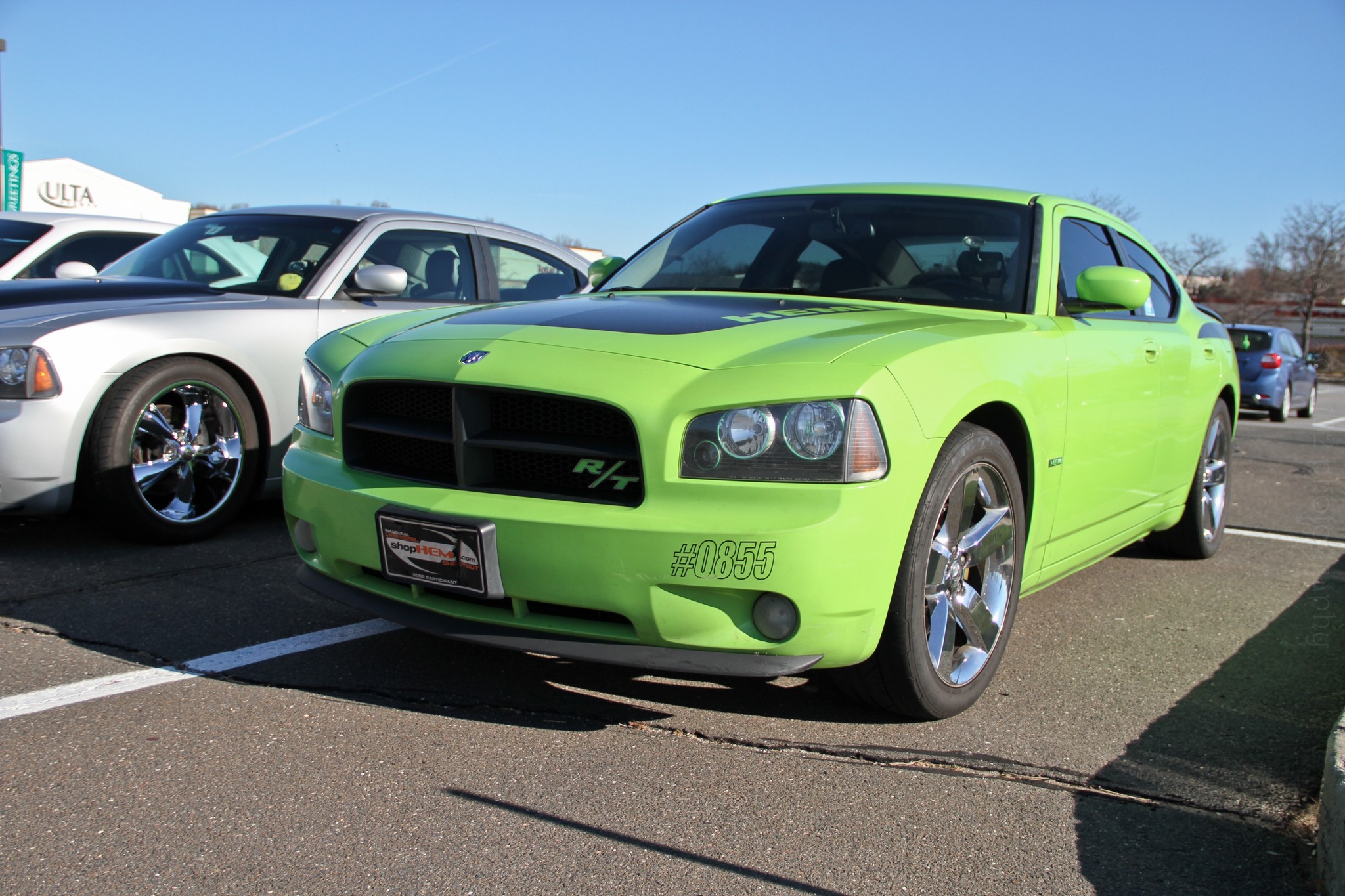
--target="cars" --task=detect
[1,212,272,294]
[1217,321,1319,420]
[279,183,1242,721]
[0,203,599,544]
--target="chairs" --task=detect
[413,249,465,304]
